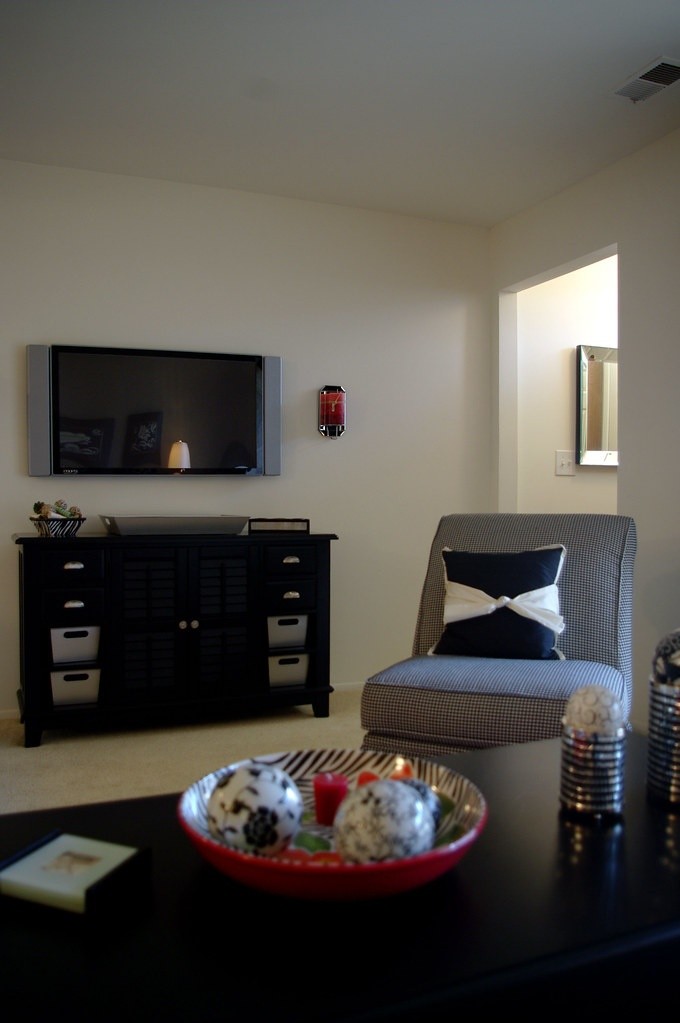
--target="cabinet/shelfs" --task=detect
[10,529,339,749]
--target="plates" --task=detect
[179,749,489,895]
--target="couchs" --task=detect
[360,514,638,758]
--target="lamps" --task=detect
[167,439,190,468]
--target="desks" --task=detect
[0,731,680,1023]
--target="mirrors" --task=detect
[575,344,618,467]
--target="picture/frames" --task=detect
[59,416,115,467]
[123,411,163,466]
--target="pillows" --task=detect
[426,541,567,660]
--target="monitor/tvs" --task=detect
[24,344,282,477]
